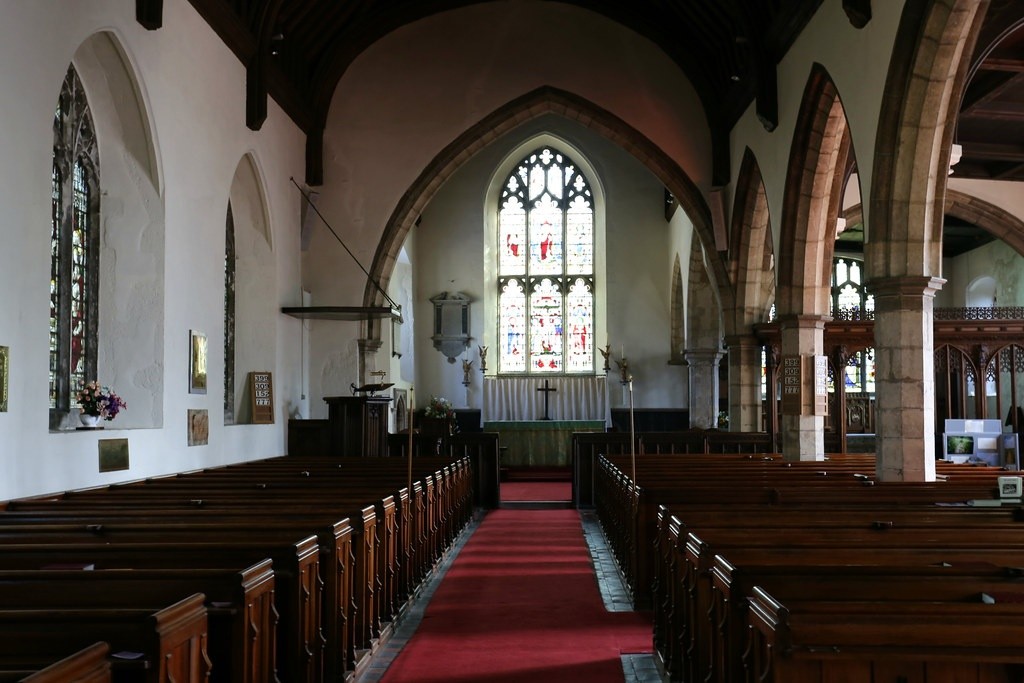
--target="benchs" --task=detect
[596,452,1024,683]
[0,455,477,683]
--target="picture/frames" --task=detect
[187,408,209,446]
[190,329,207,395]
[0,346,9,412]
[99,439,129,472]
[393,388,407,433]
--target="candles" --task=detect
[466,346,468,360]
[621,343,624,359]
[606,332,608,347]
[483,334,486,346]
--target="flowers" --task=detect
[718,411,729,421]
[75,380,126,421]
[424,394,457,420]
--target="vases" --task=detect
[79,414,101,427]
[719,418,725,423]
